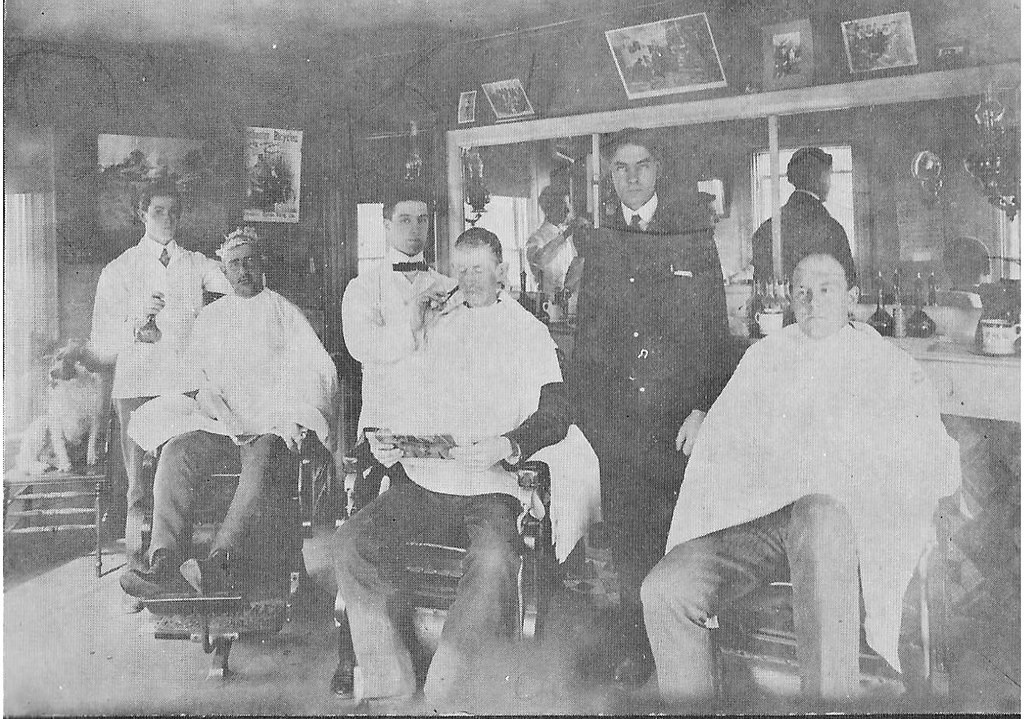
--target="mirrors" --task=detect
[446,64,1021,362]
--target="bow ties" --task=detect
[393,262,428,271]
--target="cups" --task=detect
[981,319,1021,357]
[755,312,783,336]
[543,301,562,321]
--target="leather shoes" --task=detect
[330,653,355,698]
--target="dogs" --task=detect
[18,344,106,478]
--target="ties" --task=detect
[629,214,646,270]
[159,248,171,268]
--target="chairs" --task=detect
[701,421,958,703]
[121,298,335,674]
[329,344,571,634]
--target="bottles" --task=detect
[137,296,162,344]
[749,277,798,334]
[865,269,935,341]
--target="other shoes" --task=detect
[118,556,184,598]
[180,558,227,597]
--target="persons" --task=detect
[944,236,997,287]
[639,247,956,716]
[751,147,859,296]
[525,182,590,322]
[92,177,573,709]
[464,87,528,118]
[572,125,740,692]
[774,22,908,80]
[630,35,694,81]
